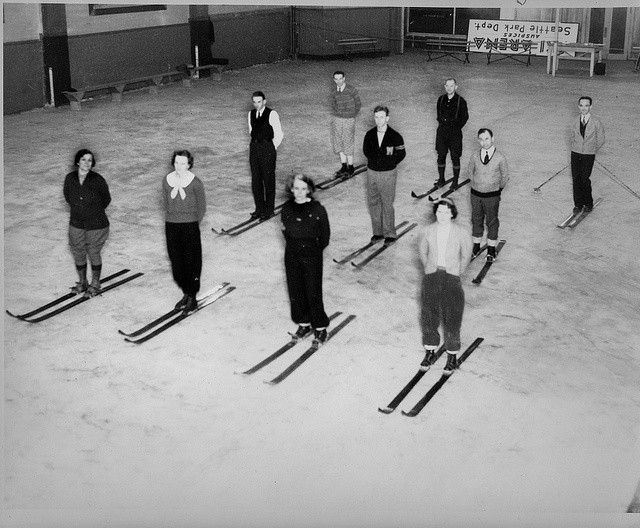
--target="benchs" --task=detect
[425,39,476,64]
[61,57,229,111]
[338,36,382,62]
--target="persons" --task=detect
[433,78,469,190]
[64,150,111,296]
[418,197,473,376]
[282,173,329,346]
[469,128,510,261]
[329,72,361,178]
[567,97,594,214]
[161,150,206,315]
[363,105,406,244]
[248,91,284,222]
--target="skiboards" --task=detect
[333,221,417,267]
[378,337,485,416]
[119,283,236,343]
[234,311,356,385]
[556,198,604,229]
[411,176,470,201]
[315,163,368,190]
[471,239,506,284]
[211,202,285,235]
[6,268,144,323]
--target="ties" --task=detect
[583,116,585,126]
[338,87,341,93]
[484,151,490,163]
[258,112,260,118]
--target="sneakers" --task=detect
[253,212,260,218]
[295,326,310,338]
[420,351,437,370]
[336,163,347,175]
[258,212,266,220]
[315,329,327,344]
[573,204,583,212]
[344,165,355,176]
[473,244,480,255]
[383,237,396,244]
[583,205,591,212]
[175,294,188,310]
[184,295,197,311]
[443,353,457,375]
[487,247,496,260]
[370,235,384,242]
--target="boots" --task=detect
[72,268,89,292]
[433,165,445,185]
[449,168,459,190]
[86,270,101,294]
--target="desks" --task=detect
[546,44,595,77]
